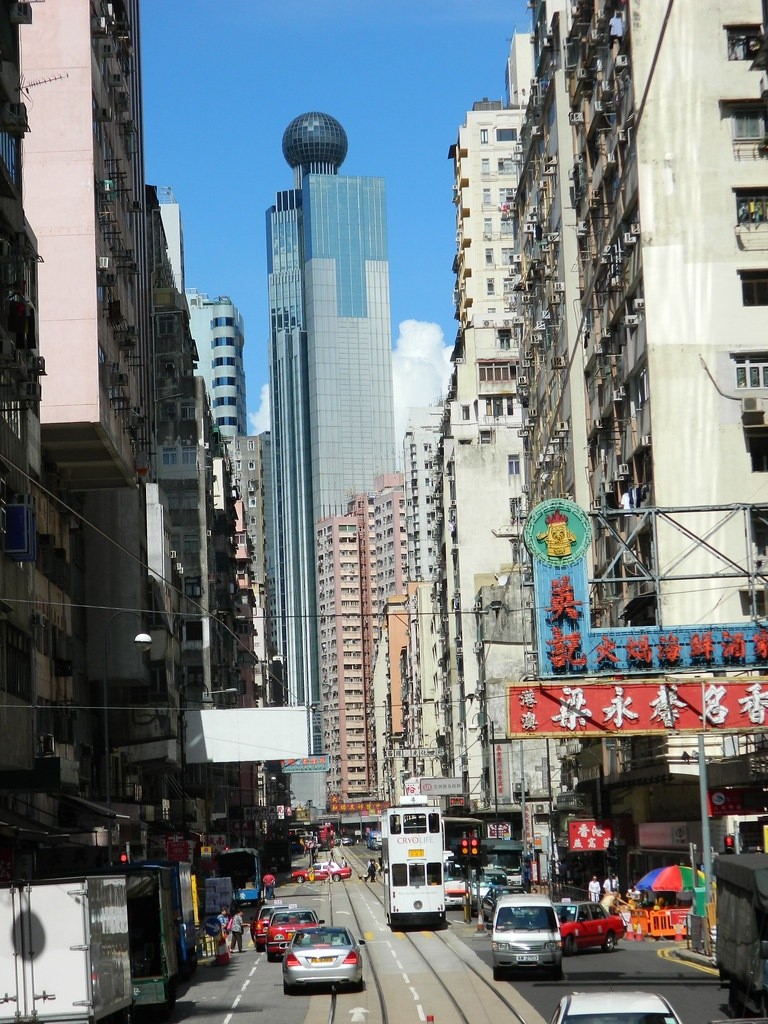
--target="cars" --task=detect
[291,862,352,883]
[550,991,682,1024]
[281,926,366,993]
[250,900,326,962]
[290,844,303,855]
[444,851,531,923]
[334,838,354,847]
[554,898,625,956]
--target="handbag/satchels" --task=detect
[241,926,244,934]
[226,917,233,931]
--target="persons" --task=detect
[588,875,601,902]
[603,873,619,892]
[342,857,346,868]
[229,910,249,952]
[216,909,229,931]
[625,884,641,901]
[263,872,275,900]
[601,891,618,912]
[325,863,333,883]
[365,859,381,882]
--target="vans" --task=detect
[491,893,563,980]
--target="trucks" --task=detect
[214,848,268,906]
[711,852,768,1018]
[0,861,199,1024]
[368,831,383,850]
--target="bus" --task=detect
[381,795,446,927]
[289,828,309,837]
[304,836,318,846]
[316,825,334,851]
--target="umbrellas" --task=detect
[637,865,718,892]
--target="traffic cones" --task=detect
[626,922,634,941]
[636,923,643,941]
[218,935,230,966]
[675,920,684,940]
[475,911,486,933]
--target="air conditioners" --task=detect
[513,782,528,793]
[533,803,550,815]
[432,0,649,775]
[1,1,238,756]
[741,396,763,416]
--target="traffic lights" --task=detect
[119,853,128,863]
[723,834,737,855]
[460,837,479,855]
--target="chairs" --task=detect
[332,936,344,945]
[300,938,311,945]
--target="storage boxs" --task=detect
[206,878,232,914]
[246,882,255,889]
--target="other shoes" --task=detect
[364,879,376,882]
